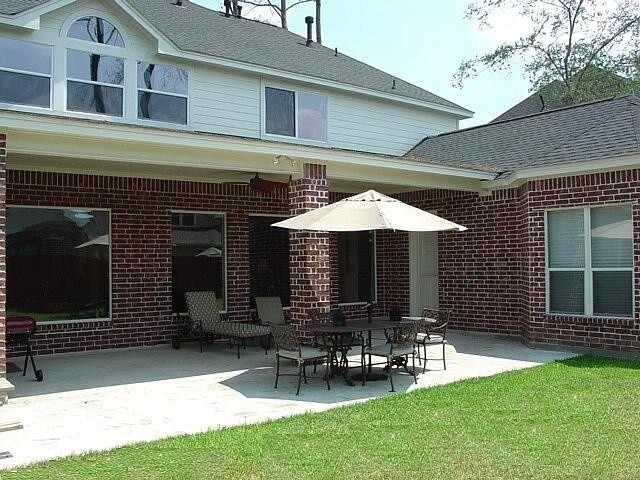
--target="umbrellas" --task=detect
[270,187,470,373]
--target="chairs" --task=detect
[171,290,287,359]
[270,307,453,395]
